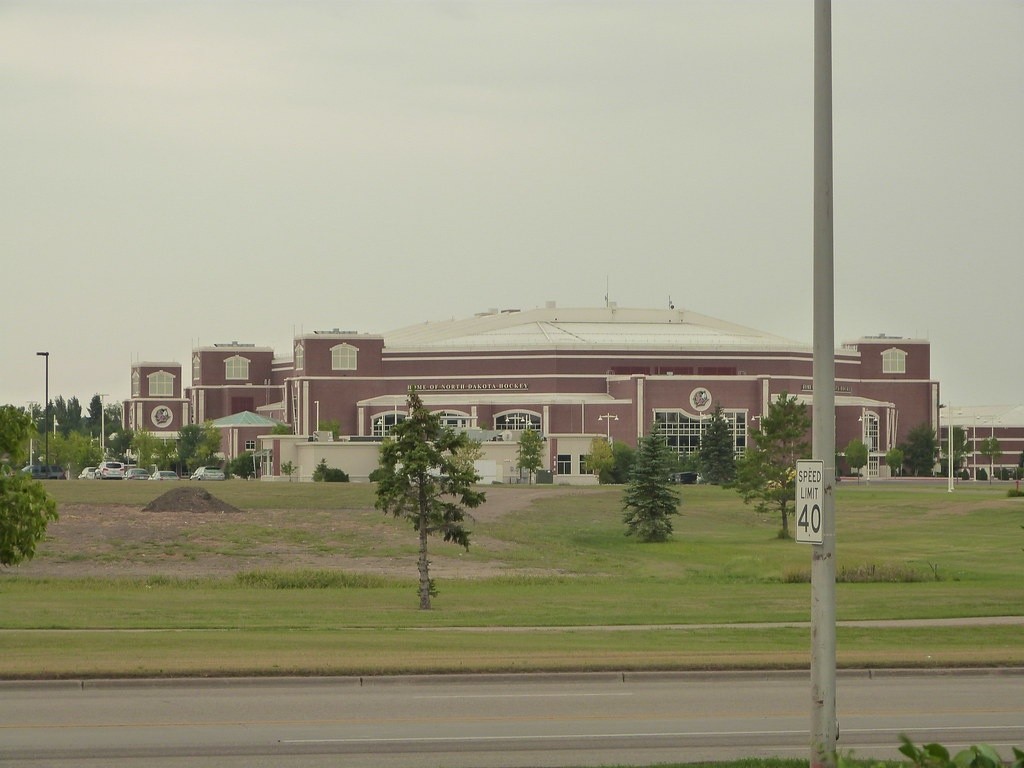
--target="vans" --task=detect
[21,465,67,480]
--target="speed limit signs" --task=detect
[794,459,825,546]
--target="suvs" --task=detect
[95,462,124,480]
[189,466,225,481]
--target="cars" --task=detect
[79,467,97,480]
[124,468,151,480]
[148,470,181,481]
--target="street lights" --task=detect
[526,415,532,431]
[939,410,1002,491]
[752,416,762,432]
[598,413,619,442]
[505,414,524,430]
[26,400,39,466]
[378,414,384,438]
[96,393,110,453]
[700,412,708,450]
[858,413,877,480]
